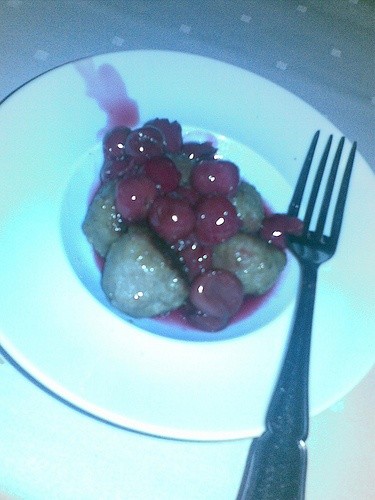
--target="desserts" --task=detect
[82,118,305,334]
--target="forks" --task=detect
[235,128,358,500]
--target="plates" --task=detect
[0,48,375,443]
